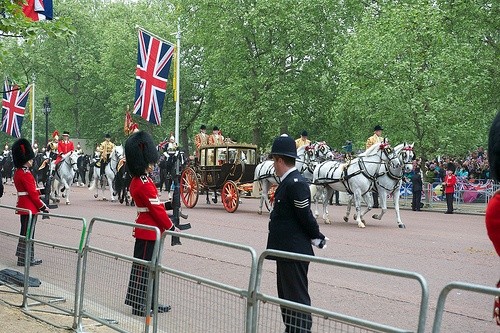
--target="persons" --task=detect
[405,164,417,182]
[295,130,311,149]
[265,137,329,333]
[485,112,500,255]
[76,144,83,154]
[46,131,59,158]
[99,134,116,176]
[411,168,422,211]
[196,125,224,158]
[366,125,385,151]
[124,132,181,316]
[426,146,490,182]
[342,140,352,153]
[49,131,74,178]
[165,135,176,151]
[93,147,100,158]
[12,139,50,267]
[1,145,10,171]
[443,163,456,214]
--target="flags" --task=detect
[2,0,53,21]
[0,79,30,139]
[133,30,174,126]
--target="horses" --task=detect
[153,146,191,202]
[86,143,125,203]
[251,140,336,218]
[0,150,102,205]
[343,141,417,230]
[309,136,403,229]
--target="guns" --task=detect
[40,158,58,219]
[168,158,191,246]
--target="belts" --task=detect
[17,192,29,195]
[136,207,150,212]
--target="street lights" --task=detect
[42,96,51,146]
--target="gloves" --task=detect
[311,236,329,250]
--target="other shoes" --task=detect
[31,258,42,265]
[132,306,153,317]
[18,257,34,266]
[152,303,171,313]
[445,210,452,214]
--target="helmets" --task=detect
[170,131,174,141]
[77,142,81,148]
[4,142,9,149]
[53,131,60,140]
[34,144,38,148]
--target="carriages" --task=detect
[180,141,417,228]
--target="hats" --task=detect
[12,139,36,169]
[268,133,301,160]
[106,134,111,138]
[213,126,219,131]
[200,125,206,130]
[62,131,70,136]
[125,132,161,178]
[445,163,456,174]
[415,168,420,172]
[374,125,383,130]
[301,131,308,136]
